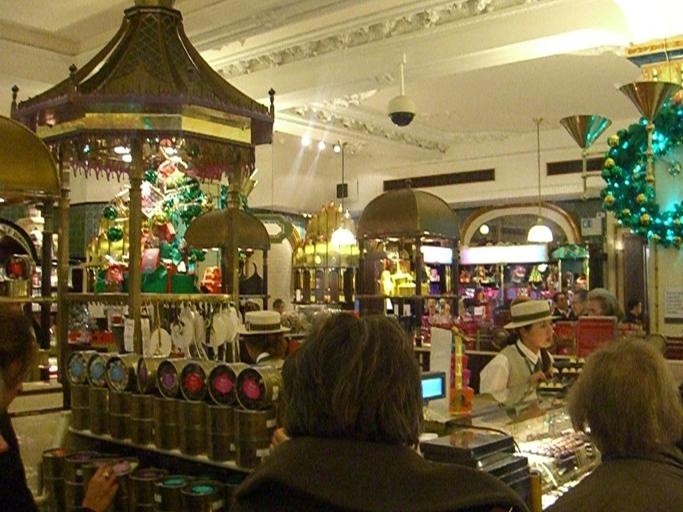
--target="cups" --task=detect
[6,279,29,299]
[111,323,124,353]
[449,386,474,416]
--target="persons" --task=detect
[542,337,683,512]
[572,288,588,321]
[552,292,579,323]
[425,297,440,317]
[480,299,564,407]
[0,301,119,512]
[234,307,294,363]
[273,299,299,326]
[466,287,489,308]
[622,296,642,323]
[586,287,625,322]
[227,313,530,512]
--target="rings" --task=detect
[103,471,110,478]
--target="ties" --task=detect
[533,356,545,374]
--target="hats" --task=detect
[500,298,567,331]
[235,308,292,337]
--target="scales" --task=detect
[419,370,516,470]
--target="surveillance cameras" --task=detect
[387,96,417,127]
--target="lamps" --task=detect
[330,137,356,251]
[559,113,614,201]
[614,80,682,191]
[523,109,554,241]
